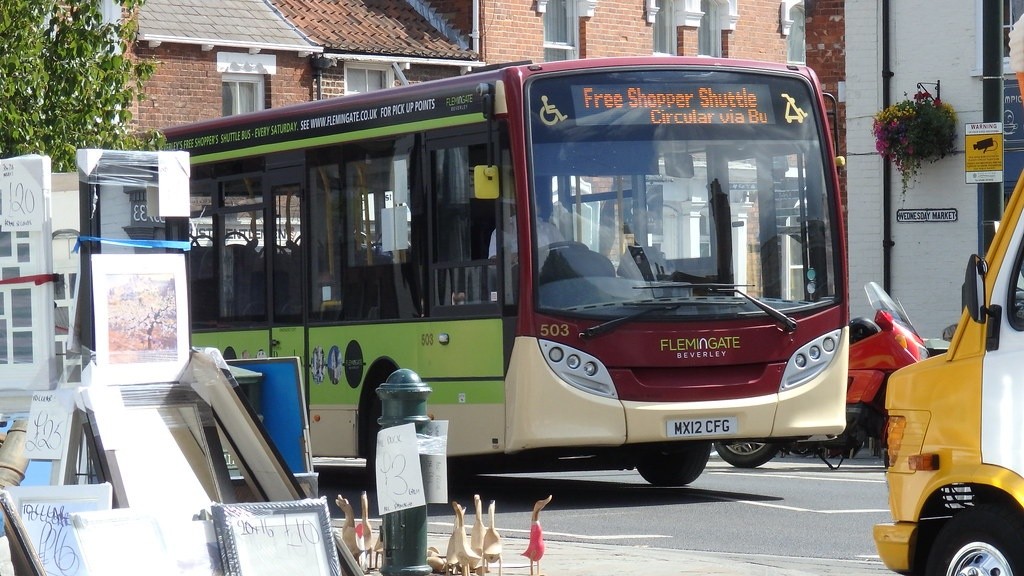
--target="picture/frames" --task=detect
[0,489,50,576]
[229,471,320,502]
[76,146,194,363]
[186,344,310,502]
[67,504,224,576]
[3,481,113,576]
[79,235,193,387]
[211,496,345,576]
[76,382,237,509]
[224,355,321,502]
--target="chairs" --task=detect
[189,227,412,327]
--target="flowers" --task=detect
[869,91,961,213]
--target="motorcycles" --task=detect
[713,281,932,468]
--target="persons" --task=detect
[488,185,568,302]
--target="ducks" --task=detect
[357,490,373,570]
[482,500,503,576]
[469,494,491,573]
[445,501,483,575]
[373,533,384,569]
[335,494,365,568]
[520,494,553,576]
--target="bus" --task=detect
[868,168,1024,576]
[127,55,853,492]
